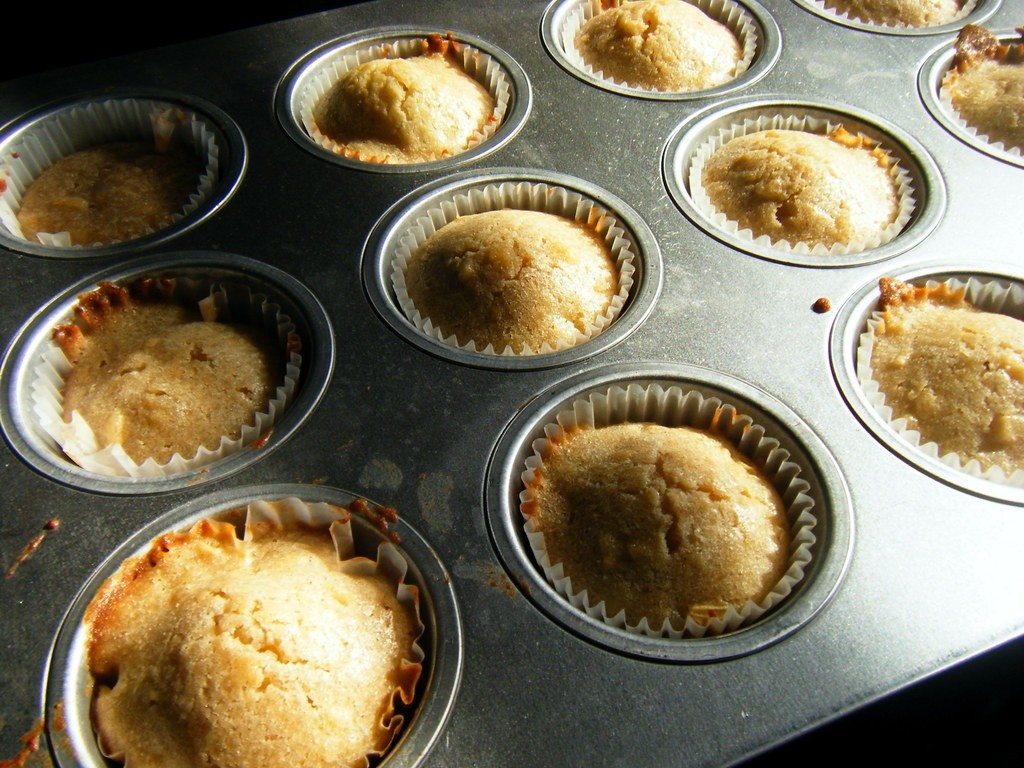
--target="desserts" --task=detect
[0,0,1024,768]
